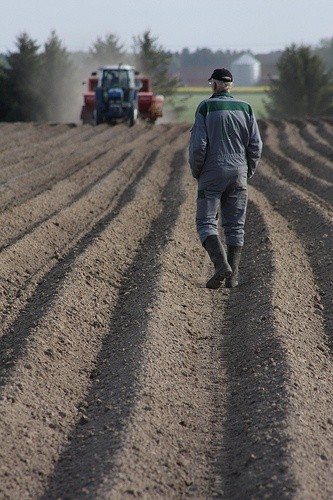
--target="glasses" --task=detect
[209,81,215,85]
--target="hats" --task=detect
[208,68,233,82]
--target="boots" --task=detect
[225,244,242,288]
[202,234,233,289]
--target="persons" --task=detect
[110,72,118,86]
[188,67,263,288]
